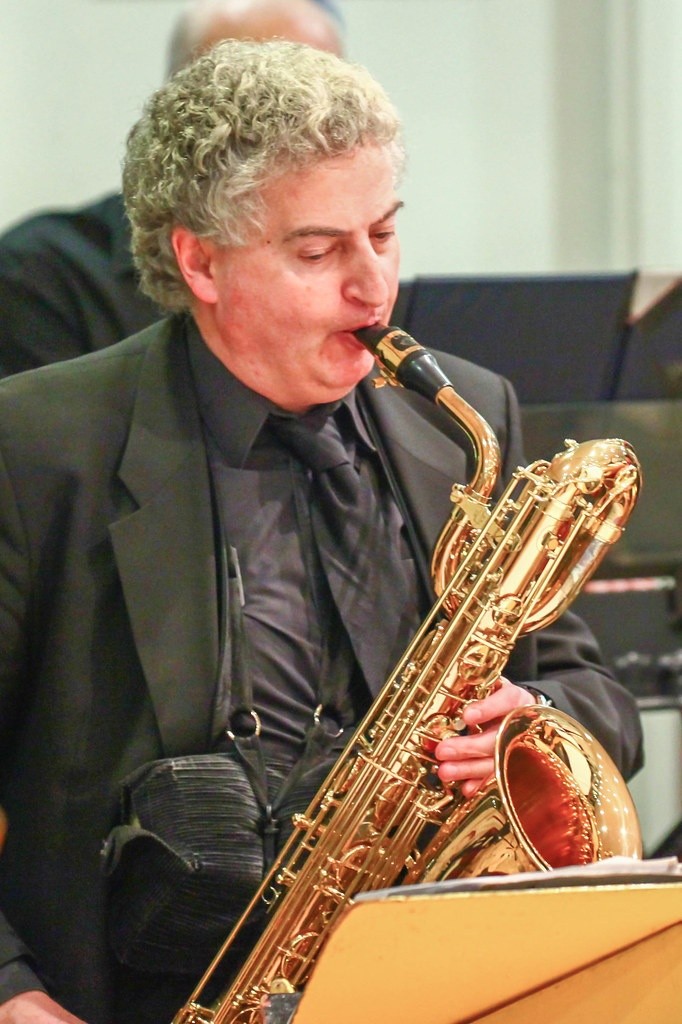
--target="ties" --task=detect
[272,404,424,702]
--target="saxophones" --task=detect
[168,321,643,1023]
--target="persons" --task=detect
[0,0,347,378]
[0,37,645,1024]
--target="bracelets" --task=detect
[518,684,553,707]
